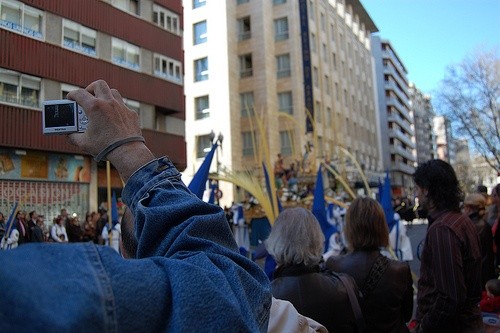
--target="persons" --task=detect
[0,209,123,254]
[413,159,486,333]
[0,80,272,333]
[223,197,270,262]
[393,194,426,221]
[264,207,370,333]
[275,154,306,205]
[326,197,414,333]
[463,182,500,313]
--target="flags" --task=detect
[380,177,395,232]
[312,170,338,255]
[188,143,217,201]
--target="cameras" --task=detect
[42,100,89,136]
